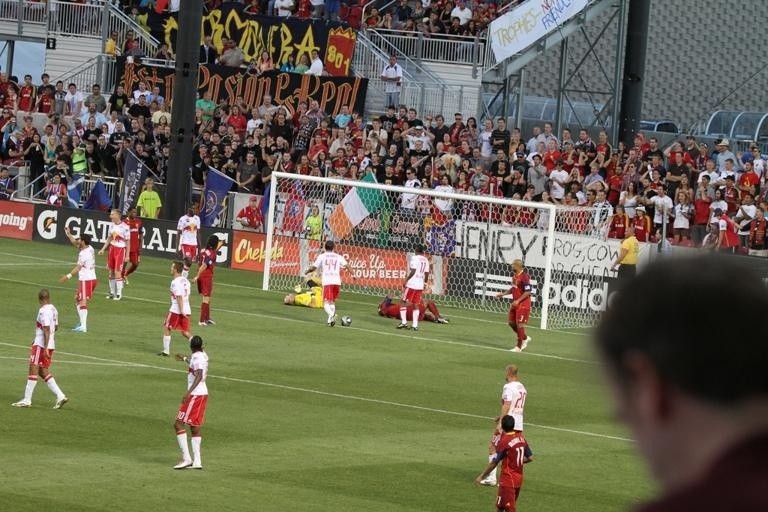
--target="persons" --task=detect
[496,259,532,354]
[97,208,145,301]
[284,240,355,328]
[0,0,768,278]
[157,207,223,357]
[378,292,450,324]
[475,416,532,512]
[479,364,527,488]
[60,226,97,335]
[592,249,768,512]
[173,335,210,470]
[395,244,430,331]
[12,289,69,409]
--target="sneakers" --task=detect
[12,399,32,408]
[479,476,497,487]
[185,464,203,469]
[72,326,87,333]
[438,318,448,324]
[509,346,522,353]
[205,319,216,325]
[397,322,408,329]
[53,397,68,410]
[106,291,115,299]
[113,292,122,301]
[198,320,207,325]
[521,336,532,350]
[173,457,193,469]
[408,326,418,331]
[157,351,170,356]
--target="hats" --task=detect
[686,135,695,140]
[635,206,646,214]
[249,195,257,201]
[714,208,722,217]
[716,139,730,146]
[516,151,524,156]
[723,175,735,181]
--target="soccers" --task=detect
[341,316,352,326]
